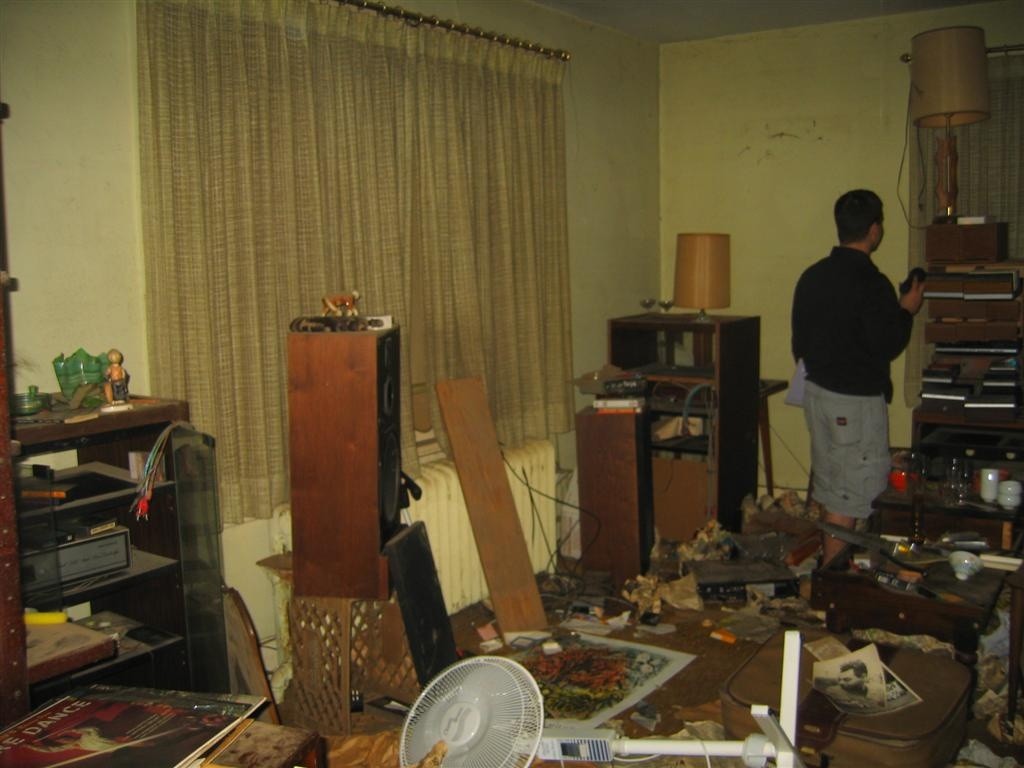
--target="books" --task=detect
[593,378,644,413]
[0,685,265,768]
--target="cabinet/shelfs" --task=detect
[606,311,760,547]
[9,392,227,696]
[811,222,1024,734]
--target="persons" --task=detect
[792,190,926,568]
[825,660,880,713]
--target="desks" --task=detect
[760,377,789,498]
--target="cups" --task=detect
[949,549,984,581]
[980,468,1000,502]
[946,453,973,502]
[996,478,1022,510]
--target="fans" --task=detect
[397,628,804,768]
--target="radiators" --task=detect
[271,439,559,704]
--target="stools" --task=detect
[723,629,973,768]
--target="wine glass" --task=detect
[639,298,655,320]
[658,300,674,314]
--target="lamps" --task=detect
[910,25,990,224]
[673,232,730,323]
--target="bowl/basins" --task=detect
[888,473,918,492]
[10,392,51,417]
